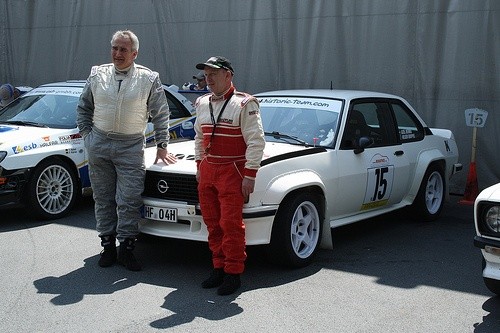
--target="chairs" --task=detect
[331,111,372,149]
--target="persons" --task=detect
[193,56,265,295]
[182,71,209,91]
[76,31,177,272]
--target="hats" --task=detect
[196,55,235,75]
[193,71,205,80]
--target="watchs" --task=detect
[157,142,167,149]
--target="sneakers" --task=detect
[201,268,242,295]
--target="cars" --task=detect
[0,80,196,219]
[138,88,464,270]
[474,181,499,301]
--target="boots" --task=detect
[98,236,142,271]
